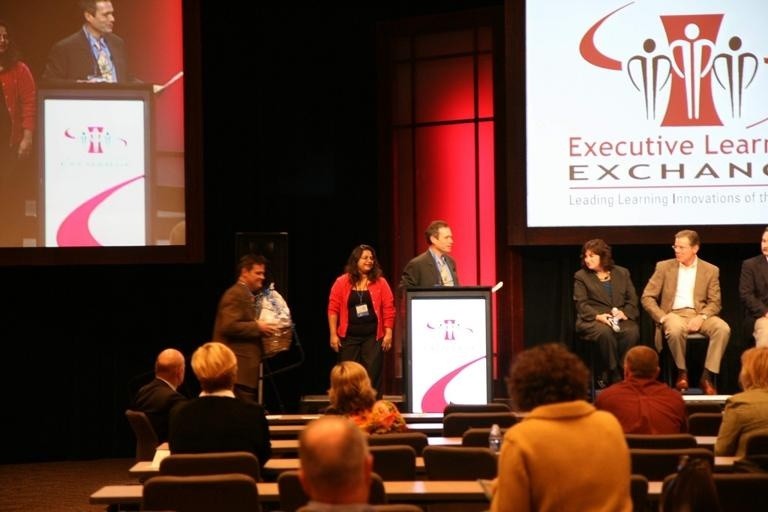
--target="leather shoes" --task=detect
[676,372,688,392]
[698,377,718,395]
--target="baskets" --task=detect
[261,322,291,354]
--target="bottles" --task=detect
[611,307,619,325]
[489,423,502,452]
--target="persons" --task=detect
[212,253,279,402]
[640,229,731,394]
[738,226,768,347]
[572,239,641,389]
[397,220,459,289]
[490,344,767,511]
[299,361,410,511]
[38,0,164,96]
[0,21,37,247]
[136,343,273,483]
[326,244,396,401]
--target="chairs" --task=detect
[663,331,718,395]
[87,389,767,511]
[576,337,608,402]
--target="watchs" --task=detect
[700,315,707,320]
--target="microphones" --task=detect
[86,55,113,81]
[435,268,456,286]
[607,316,621,333]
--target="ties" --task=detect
[437,259,449,286]
[95,39,112,81]
